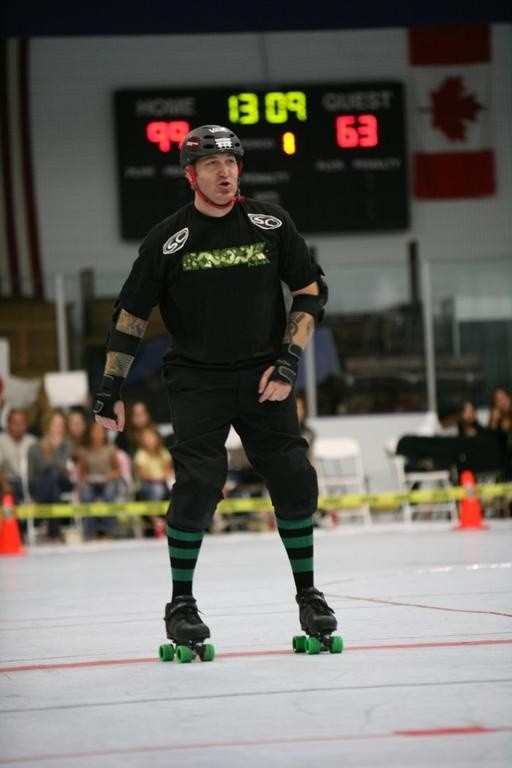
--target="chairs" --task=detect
[17,429,459,550]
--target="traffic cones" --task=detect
[0,491,29,557]
[450,469,491,534]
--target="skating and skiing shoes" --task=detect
[159,594,214,663]
[293,587,343,654]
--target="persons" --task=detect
[0,393,175,543]
[91,124,339,644]
[411,387,512,519]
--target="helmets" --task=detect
[180,125,244,170]
